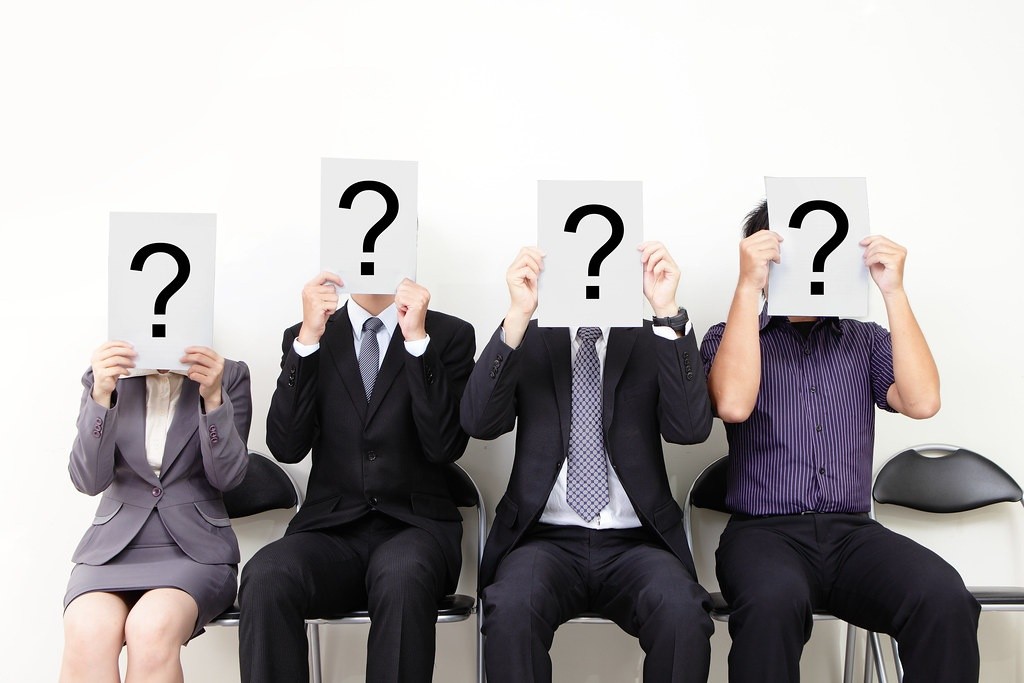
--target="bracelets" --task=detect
[653,308,689,332]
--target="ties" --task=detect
[565,327,611,523]
[358,317,384,407]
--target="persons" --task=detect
[57,340,253,683]
[700,203,982,682]
[238,273,478,683]
[459,245,713,682]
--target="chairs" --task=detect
[864,443,1024,683]
[685,455,888,683]
[168,446,323,683]
[304,461,484,683]
